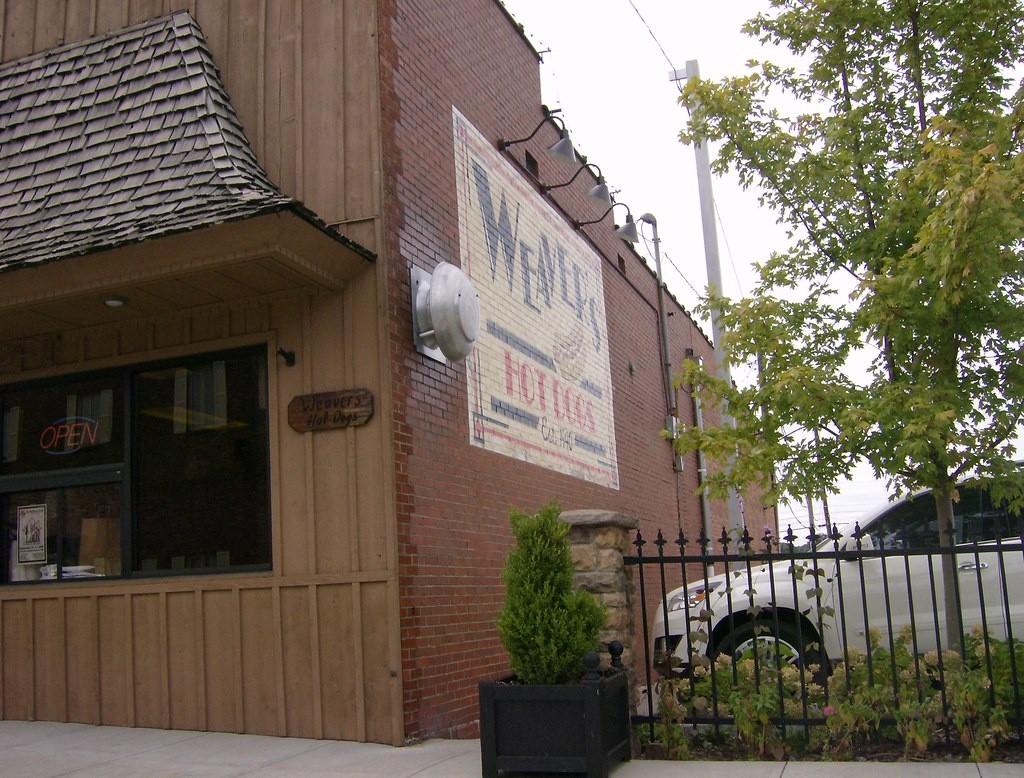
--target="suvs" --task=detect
[652,458,1024,697]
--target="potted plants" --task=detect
[478,498,632,778]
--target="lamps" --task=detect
[573,203,639,244]
[542,164,611,207]
[498,116,576,162]
